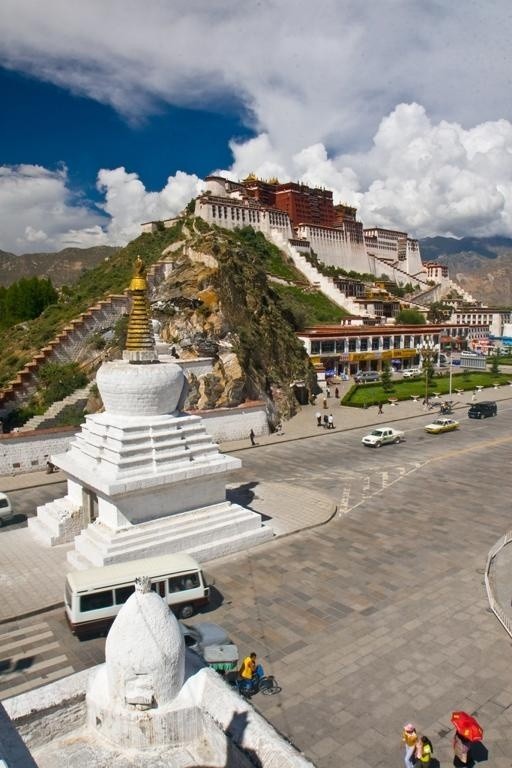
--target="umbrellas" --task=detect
[451,710,483,743]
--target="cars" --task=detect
[440,343,511,365]
[179,620,232,658]
[423,418,459,435]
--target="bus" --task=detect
[64,552,211,638]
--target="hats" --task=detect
[403,724,414,732]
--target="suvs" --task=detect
[403,368,419,378]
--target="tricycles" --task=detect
[203,643,278,697]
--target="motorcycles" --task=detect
[440,404,450,415]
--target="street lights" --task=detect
[448,334,458,397]
[416,335,439,407]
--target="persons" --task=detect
[377,400,384,415]
[249,428,256,445]
[423,400,449,415]
[471,389,476,401]
[401,724,483,768]
[310,387,340,429]
[239,652,256,699]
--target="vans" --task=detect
[354,371,380,382]
[0,491,13,529]
[467,401,496,419]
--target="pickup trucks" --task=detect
[361,427,404,449]
